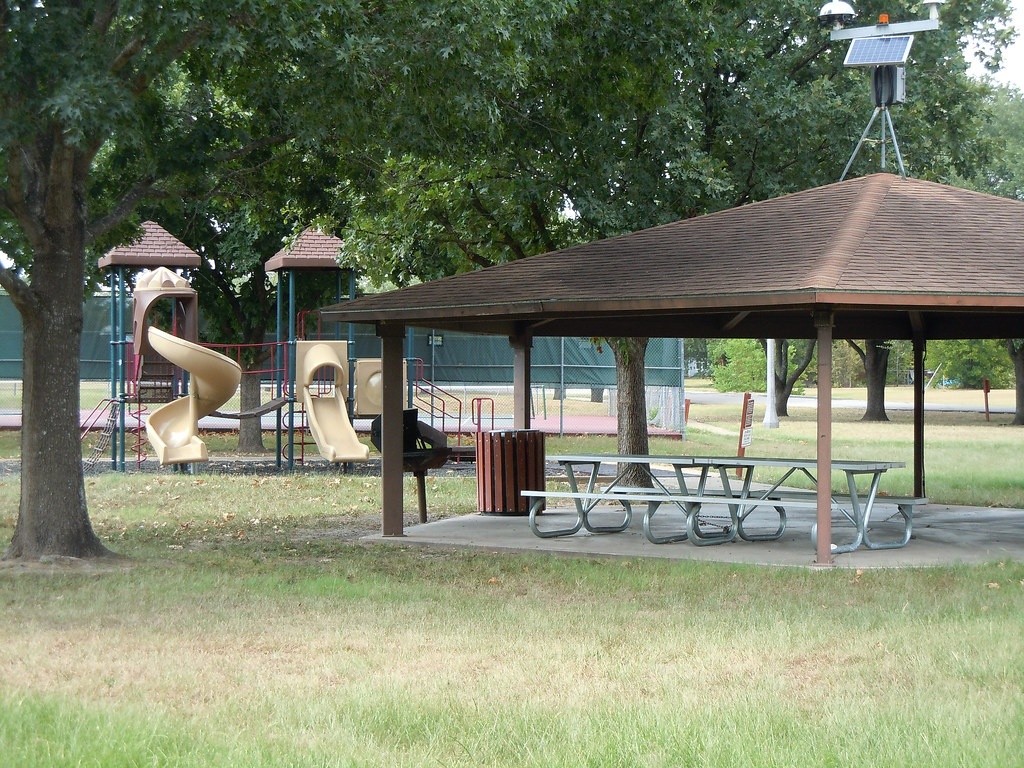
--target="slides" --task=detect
[303,385,371,461]
[145,325,242,465]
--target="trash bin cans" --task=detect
[474,429,546,516]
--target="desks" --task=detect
[692,453,907,490]
[544,453,694,491]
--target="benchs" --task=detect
[669,495,868,553]
[738,491,929,550]
[600,486,738,546]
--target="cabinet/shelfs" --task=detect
[518,490,693,544]
